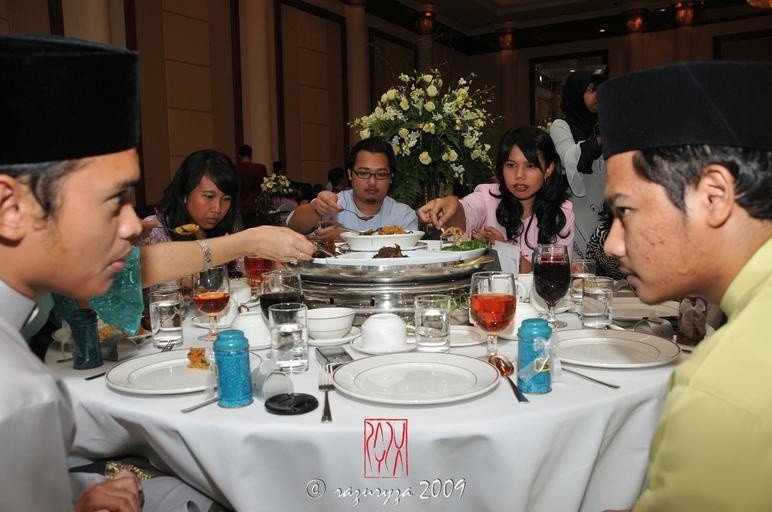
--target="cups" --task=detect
[149,289,182,348]
[363,314,416,349]
[416,294,452,351]
[268,300,309,374]
[479,273,534,302]
[574,259,596,299]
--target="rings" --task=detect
[290,257,297,265]
[295,250,303,260]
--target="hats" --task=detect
[0,32,143,168]
[592,57,772,163]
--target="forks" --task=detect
[318,364,336,423]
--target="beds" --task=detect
[39,240,714,512]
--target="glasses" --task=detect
[352,167,392,181]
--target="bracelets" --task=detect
[194,237,213,274]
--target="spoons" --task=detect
[491,351,528,402]
[154,224,200,236]
[342,208,375,220]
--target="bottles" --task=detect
[215,328,254,408]
[516,318,555,393]
[69,309,102,369]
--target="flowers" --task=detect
[260,172,296,215]
[342,60,497,209]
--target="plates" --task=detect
[449,322,484,348]
[51,325,149,343]
[306,326,363,345]
[350,333,417,353]
[572,275,685,326]
[331,350,501,407]
[314,231,486,264]
[104,348,264,395]
[558,328,681,369]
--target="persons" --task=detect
[127,146,248,324]
[1,31,227,512]
[548,68,608,261]
[285,136,419,242]
[22,223,318,337]
[236,145,344,229]
[414,122,576,275]
[595,62,771,512]
[584,201,630,281]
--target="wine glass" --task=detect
[533,245,573,328]
[187,252,304,359]
[469,273,516,375]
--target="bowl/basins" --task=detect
[299,306,355,340]
